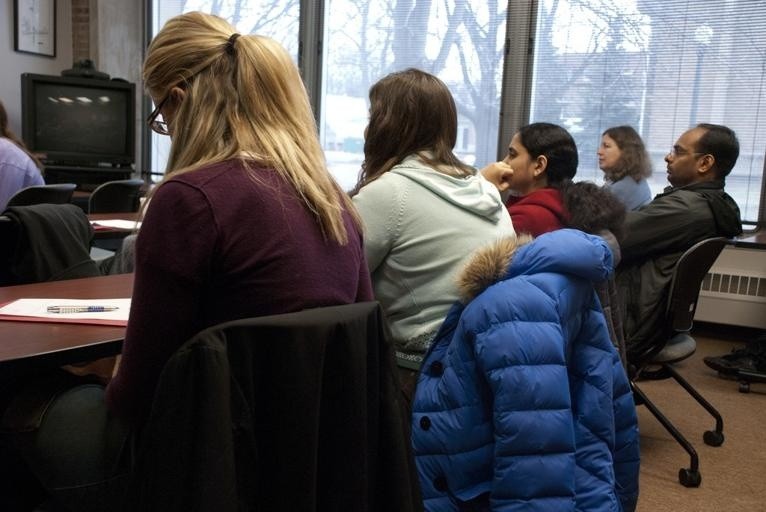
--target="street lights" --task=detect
[688,22,714,129]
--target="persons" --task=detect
[348,67,515,385]
[489,119,611,242]
[615,123,743,354]
[596,126,650,218]
[0,102,45,215]
[101,14,374,419]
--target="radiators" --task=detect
[690,245,766,329]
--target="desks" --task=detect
[0,271,135,377]
[73,192,153,214]
[86,212,142,239]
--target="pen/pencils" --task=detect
[46,305,120,314]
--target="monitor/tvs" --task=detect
[21,72,136,194]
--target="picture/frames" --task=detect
[13,0,57,60]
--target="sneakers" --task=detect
[702,345,766,379]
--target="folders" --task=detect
[0,297,131,327]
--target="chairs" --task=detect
[626,236,731,487]
[7,182,78,212]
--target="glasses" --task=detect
[147,97,170,134]
[670,149,704,157]
